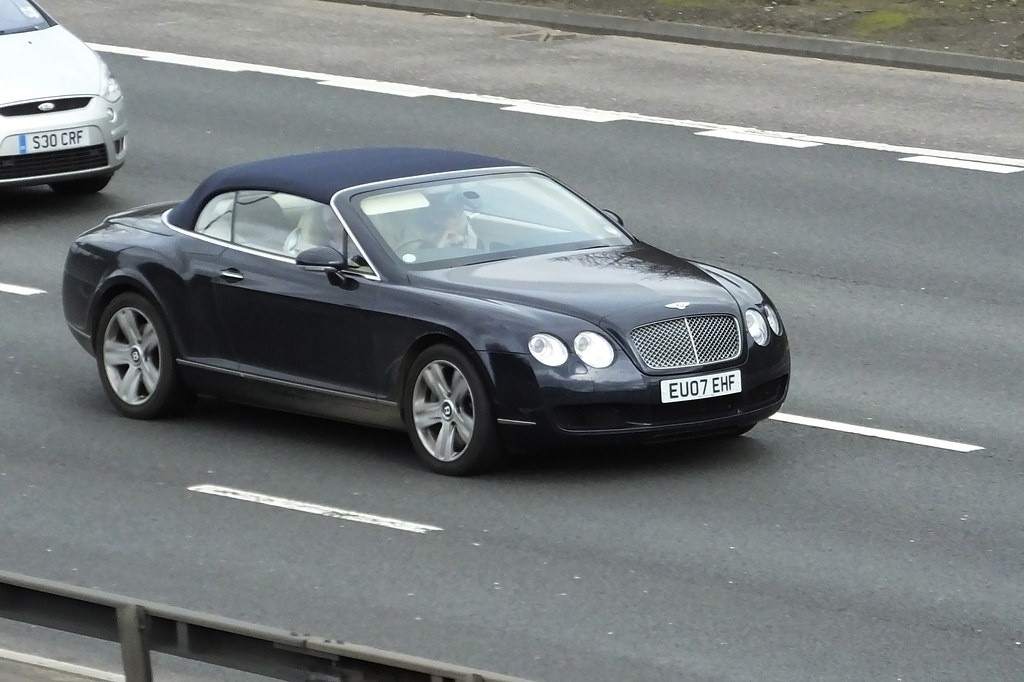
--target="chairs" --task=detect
[216,194,477,270]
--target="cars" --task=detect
[0,0,128,197]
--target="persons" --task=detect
[324,205,487,270]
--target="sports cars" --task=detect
[61,145,794,476]
